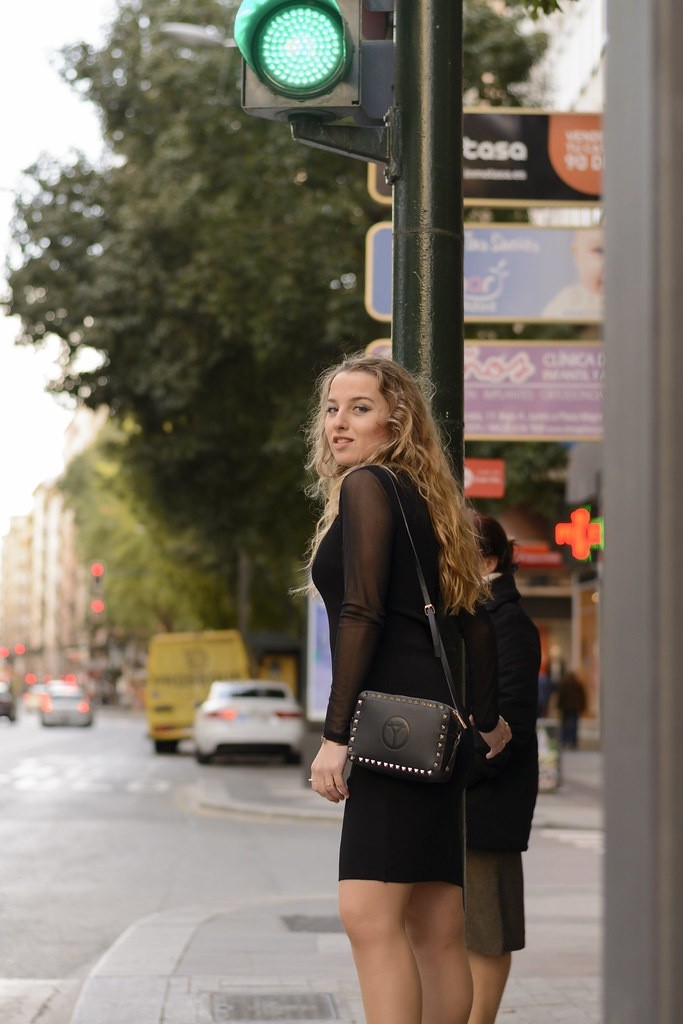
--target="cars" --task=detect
[0,675,94,729]
[192,678,310,766]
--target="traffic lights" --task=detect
[235,1,364,126]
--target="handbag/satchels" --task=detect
[346,690,463,784]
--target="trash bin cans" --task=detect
[538,717,562,794]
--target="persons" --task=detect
[308,352,541,1024]
[538,643,589,751]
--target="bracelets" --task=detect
[320,735,347,746]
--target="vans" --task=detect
[139,631,248,755]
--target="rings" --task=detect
[325,783,331,785]
[308,779,316,781]
